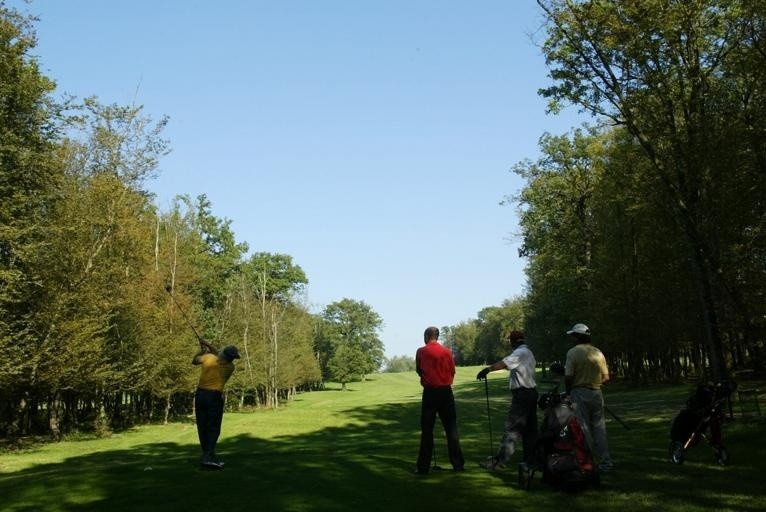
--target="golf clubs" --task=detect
[165,285,211,353]
[482,375,496,472]
[433,444,441,471]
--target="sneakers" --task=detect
[487,455,507,468]
[200,456,225,471]
[598,463,613,472]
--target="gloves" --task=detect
[477,368,489,380]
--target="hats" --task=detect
[223,346,240,359]
[507,331,524,339]
[566,323,591,336]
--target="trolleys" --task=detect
[668,363,738,465]
[519,380,600,494]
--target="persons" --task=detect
[411,326,465,473]
[565,323,616,472]
[476,331,540,472]
[191,338,240,467]
